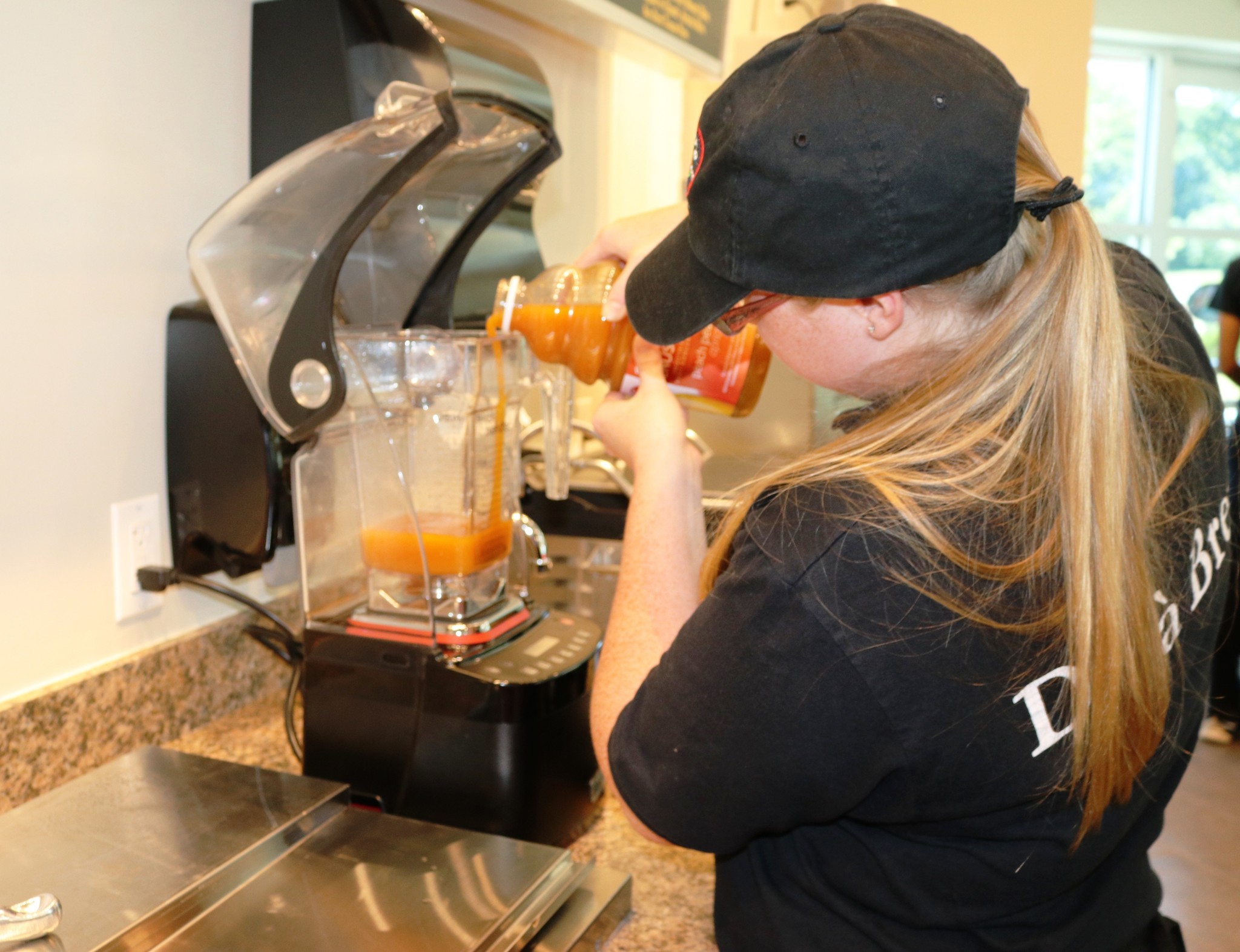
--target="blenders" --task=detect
[187,88,618,846]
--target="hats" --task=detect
[624,6,1083,344]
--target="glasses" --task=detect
[711,293,791,337]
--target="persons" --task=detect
[578,3,1240,952]
[1198,260,1240,743]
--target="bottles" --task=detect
[497,259,772,421]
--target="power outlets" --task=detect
[112,494,165,623]
